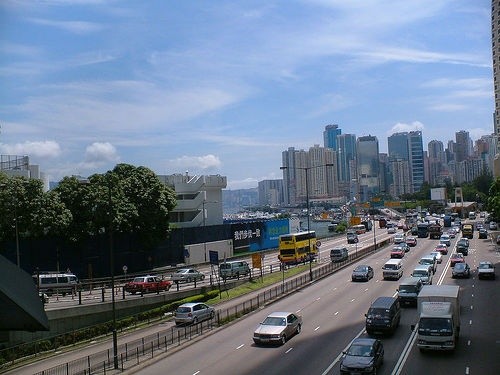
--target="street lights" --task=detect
[280,163,335,281]
[77,176,159,370]
[358,190,386,251]
[2,180,30,269]
[202,201,218,263]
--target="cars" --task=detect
[435,244,448,255]
[125,275,171,295]
[338,338,384,375]
[451,263,470,279]
[253,311,303,347]
[410,265,433,285]
[418,255,437,275]
[171,268,205,284]
[351,264,374,282]
[477,261,496,280]
[328,206,500,253]
[391,247,405,258]
[174,303,215,326]
[450,253,465,266]
[430,252,443,264]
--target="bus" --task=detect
[32,274,83,296]
[278,230,318,266]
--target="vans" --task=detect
[364,296,400,334]
[396,277,422,306]
[217,260,250,279]
[381,259,403,280]
[330,248,348,262]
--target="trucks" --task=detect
[409,285,461,353]
